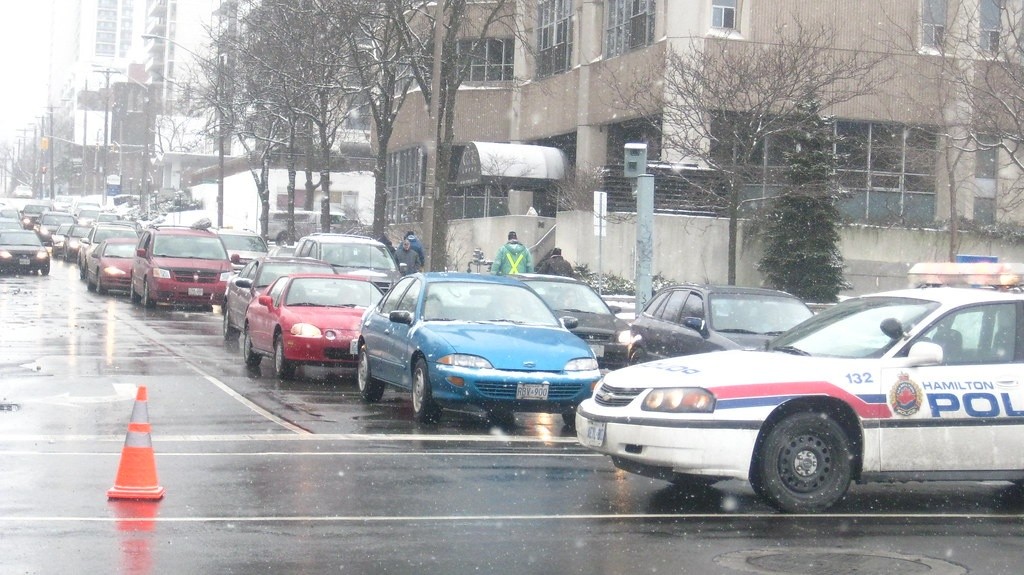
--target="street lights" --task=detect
[141,33,224,228]
[90,63,149,221]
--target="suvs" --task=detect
[130,225,239,311]
[79,223,138,280]
[210,228,273,263]
[296,232,401,294]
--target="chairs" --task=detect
[932,328,964,365]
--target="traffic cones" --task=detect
[106,385,165,501]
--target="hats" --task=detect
[508,232,517,240]
[551,248,562,256]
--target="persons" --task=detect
[537,247,575,277]
[379,230,425,275]
[491,231,534,275]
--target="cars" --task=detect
[72,206,103,225]
[576,253,1024,517]
[0,229,50,277]
[85,238,141,293]
[52,195,75,211]
[62,225,93,263]
[91,213,121,224]
[630,285,814,367]
[356,269,602,428]
[242,275,384,379]
[223,256,338,343]
[12,186,33,199]
[503,274,631,372]
[20,204,54,230]
[0,208,23,231]
[51,224,75,259]
[34,211,78,247]
